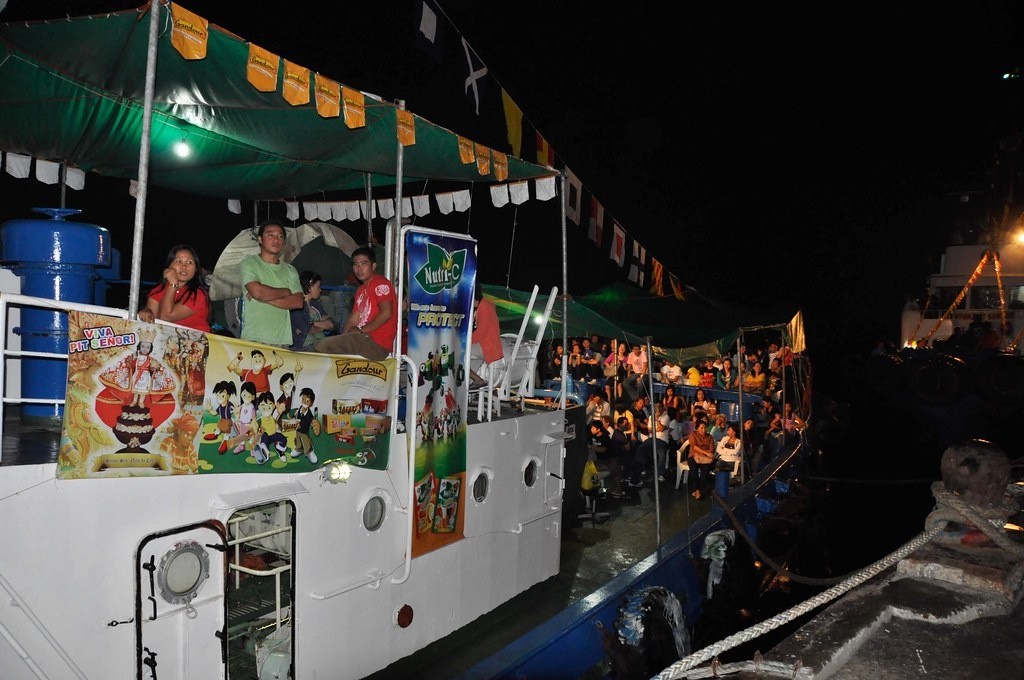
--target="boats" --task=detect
[0,0,809,680]
[902,242,1024,358]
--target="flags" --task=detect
[420,0,693,299]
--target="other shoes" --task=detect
[611,490,626,499]
[620,475,644,488]
[691,490,702,501]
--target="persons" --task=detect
[290,271,335,353]
[314,247,398,361]
[139,244,211,332]
[549,334,805,502]
[239,221,303,349]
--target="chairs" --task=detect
[731,458,742,479]
[673,439,689,489]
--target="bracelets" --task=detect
[168,284,179,288]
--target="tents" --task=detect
[0,0,564,241]
[475,272,789,361]
